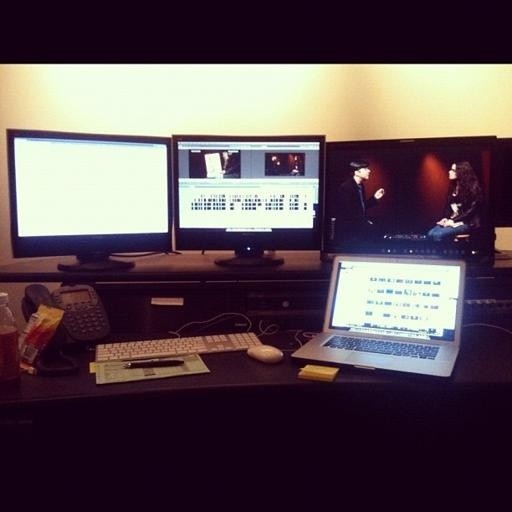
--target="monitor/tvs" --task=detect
[171,133,327,269]
[320,135,498,267]
[6,127,171,272]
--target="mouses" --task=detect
[247,344,287,368]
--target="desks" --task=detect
[0,255,512,511]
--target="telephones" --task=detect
[21,283,111,350]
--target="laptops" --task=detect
[290,252,467,381]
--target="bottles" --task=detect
[0,291,21,382]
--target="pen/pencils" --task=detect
[123,360,184,369]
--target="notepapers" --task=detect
[297,364,340,382]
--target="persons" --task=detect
[427,157,490,261]
[328,159,387,254]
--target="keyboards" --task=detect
[94,331,262,363]
